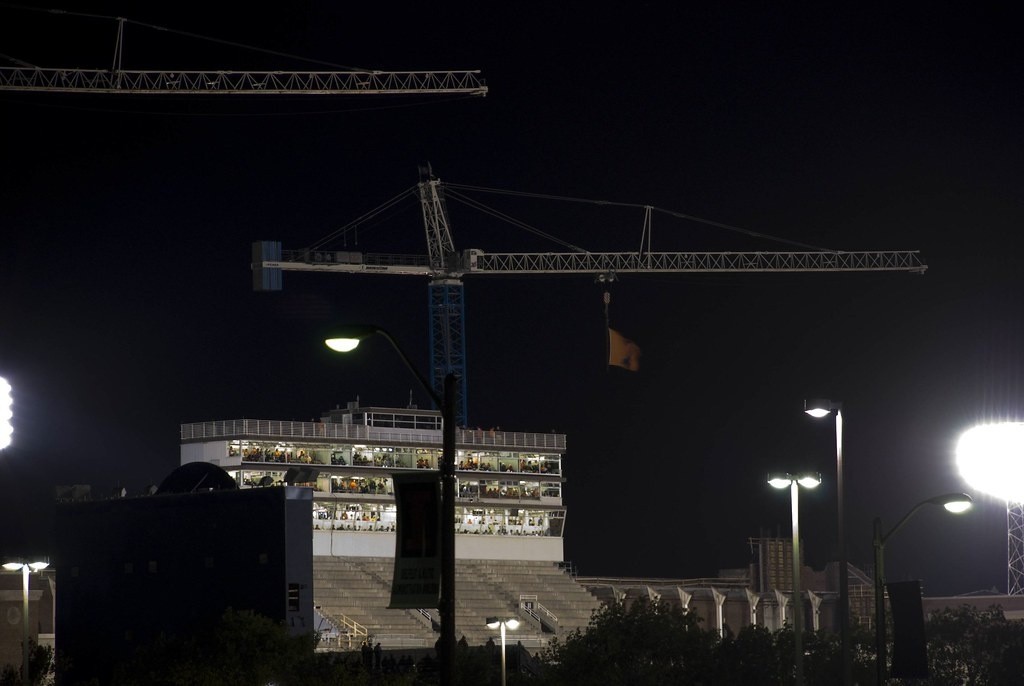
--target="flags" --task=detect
[607,327,643,372]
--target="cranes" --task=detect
[2,12,929,426]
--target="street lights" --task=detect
[1,555,50,686]
[871,493,973,686]
[323,323,459,686]
[805,396,854,686]
[767,466,824,686]
[485,615,522,686]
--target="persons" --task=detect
[229,444,552,537]
[322,635,541,680]
